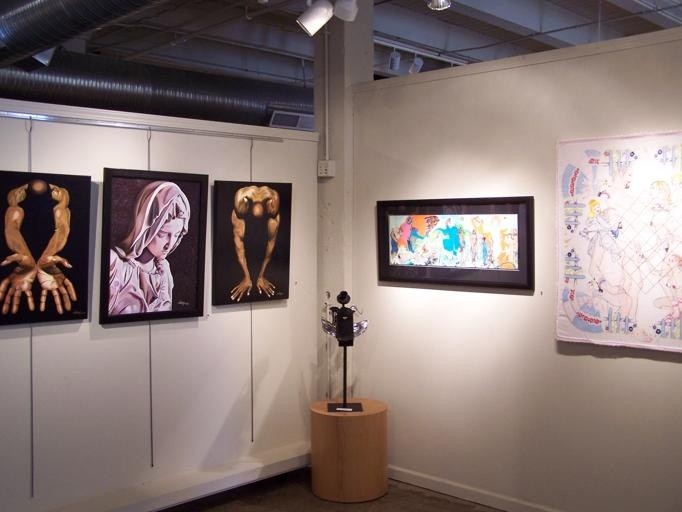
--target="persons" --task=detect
[230,185,281,302]
[0,178,78,315]
[108,181,193,316]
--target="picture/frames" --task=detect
[209,175,295,309]
[374,194,536,292]
[97,165,211,327]
[0,168,94,328]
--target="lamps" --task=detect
[294,1,362,36]
[424,0,452,16]
[383,45,424,77]
